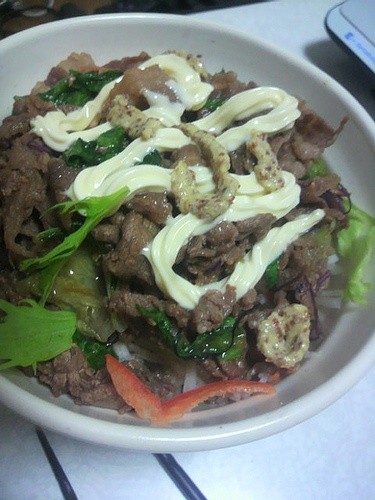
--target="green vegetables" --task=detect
[0,72,375,374]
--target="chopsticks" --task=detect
[33,427,207,500]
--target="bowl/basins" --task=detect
[0,13,375,453]
